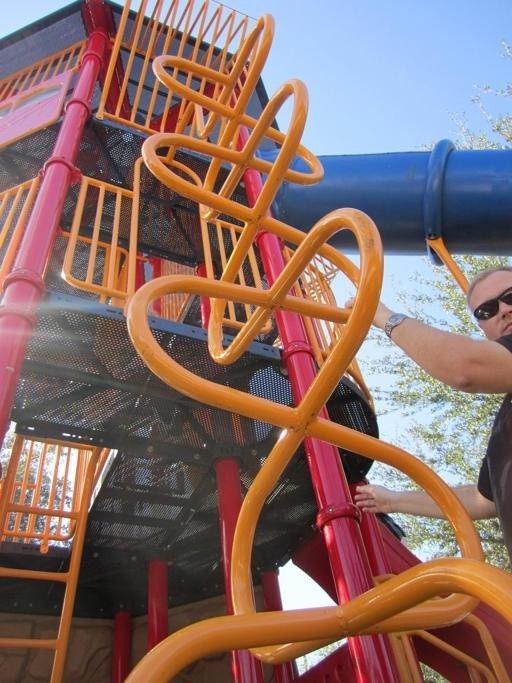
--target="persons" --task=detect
[346,264,512,564]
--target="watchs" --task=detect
[385,312,408,337]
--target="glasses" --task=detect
[473,286,512,321]
